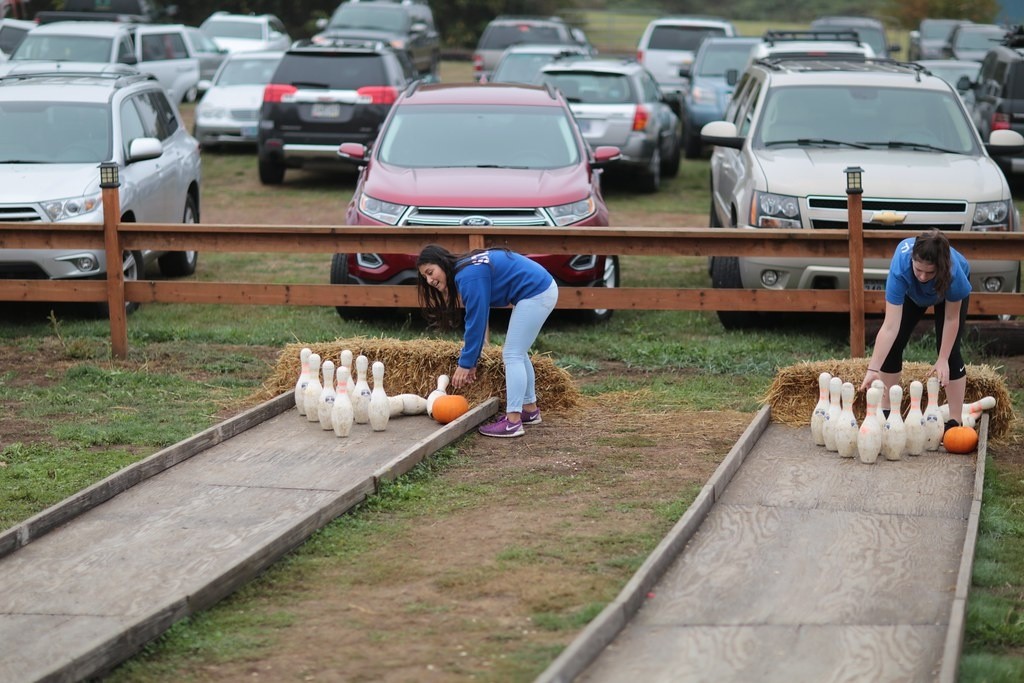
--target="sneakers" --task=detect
[478,420,525,437]
[882,409,891,417]
[497,408,542,424]
[940,419,963,446]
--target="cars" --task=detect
[192,50,287,145]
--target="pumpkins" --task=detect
[943,426,979,454]
[432,394,470,422]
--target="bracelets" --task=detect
[867,369,879,373]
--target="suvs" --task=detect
[255,38,440,185]
[330,80,622,325]
[1,70,202,319]
[699,54,1024,332]
[0,0,1024,192]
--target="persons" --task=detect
[860,232,972,446]
[416,246,558,437]
